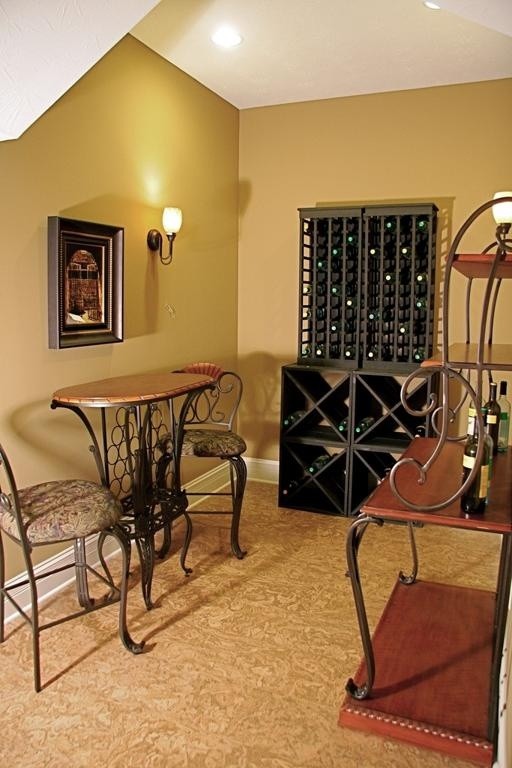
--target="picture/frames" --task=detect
[48,215,123,349]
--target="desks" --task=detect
[50,371,215,610]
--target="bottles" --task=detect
[457,416,489,515]
[466,380,486,433]
[482,382,500,456]
[496,381,511,452]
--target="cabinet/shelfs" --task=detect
[278,203,439,527]
[337,202,512,768]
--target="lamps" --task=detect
[147,206,183,265]
[492,190,512,252]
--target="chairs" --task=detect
[0,444,146,692]
[154,363,248,560]
